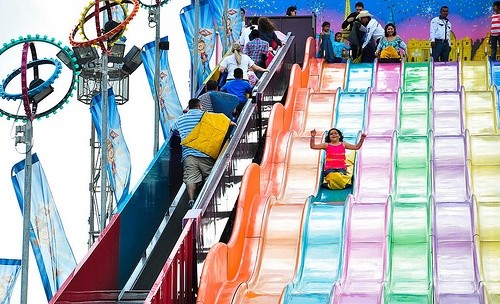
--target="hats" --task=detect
[356,10,372,19]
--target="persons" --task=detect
[170,98,238,207]
[318,2,408,63]
[220,67,254,114]
[183,80,236,117]
[241,8,245,18]
[218,42,270,83]
[430,6,451,62]
[258,17,286,68]
[244,30,269,79]
[490,1,500,60]
[238,19,258,46]
[310,128,366,189]
[286,6,297,16]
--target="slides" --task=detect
[197,37,500,304]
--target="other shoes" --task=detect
[188,199,195,208]
